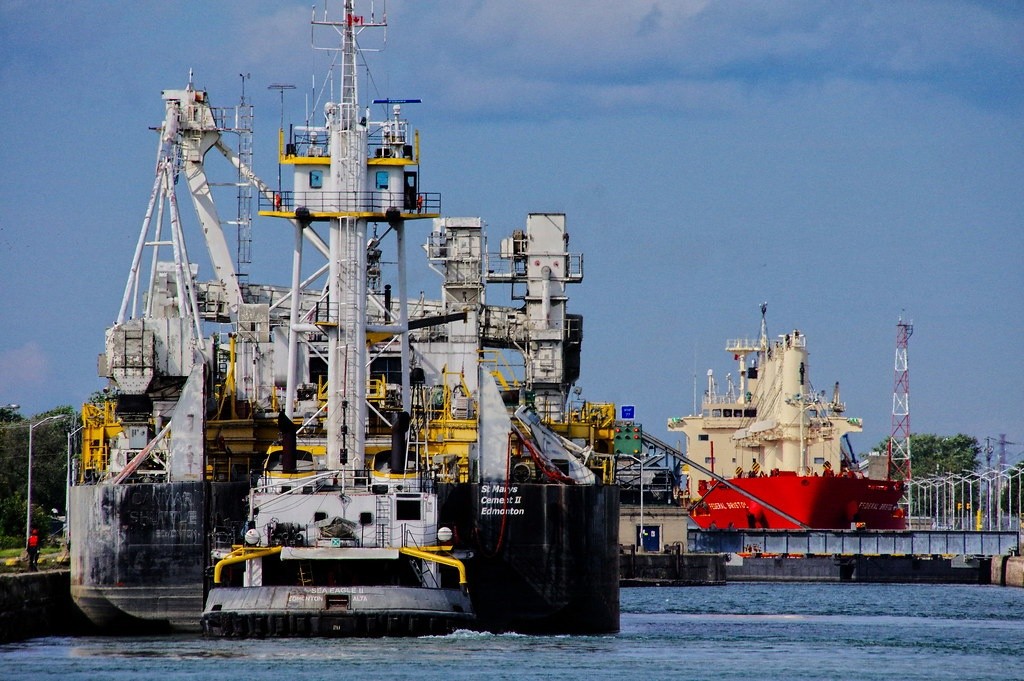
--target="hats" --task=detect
[32,529,38,534]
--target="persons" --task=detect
[26,529,38,571]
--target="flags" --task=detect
[348,14,363,25]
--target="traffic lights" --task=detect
[966,503,970,510]
[957,503,962,510]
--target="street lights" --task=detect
[65,424,84,545]
[904,462,1024,532]
[0,404,20,409]
[25,415,65,551]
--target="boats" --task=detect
[63,1,623,642]
[666,301,907,530]
